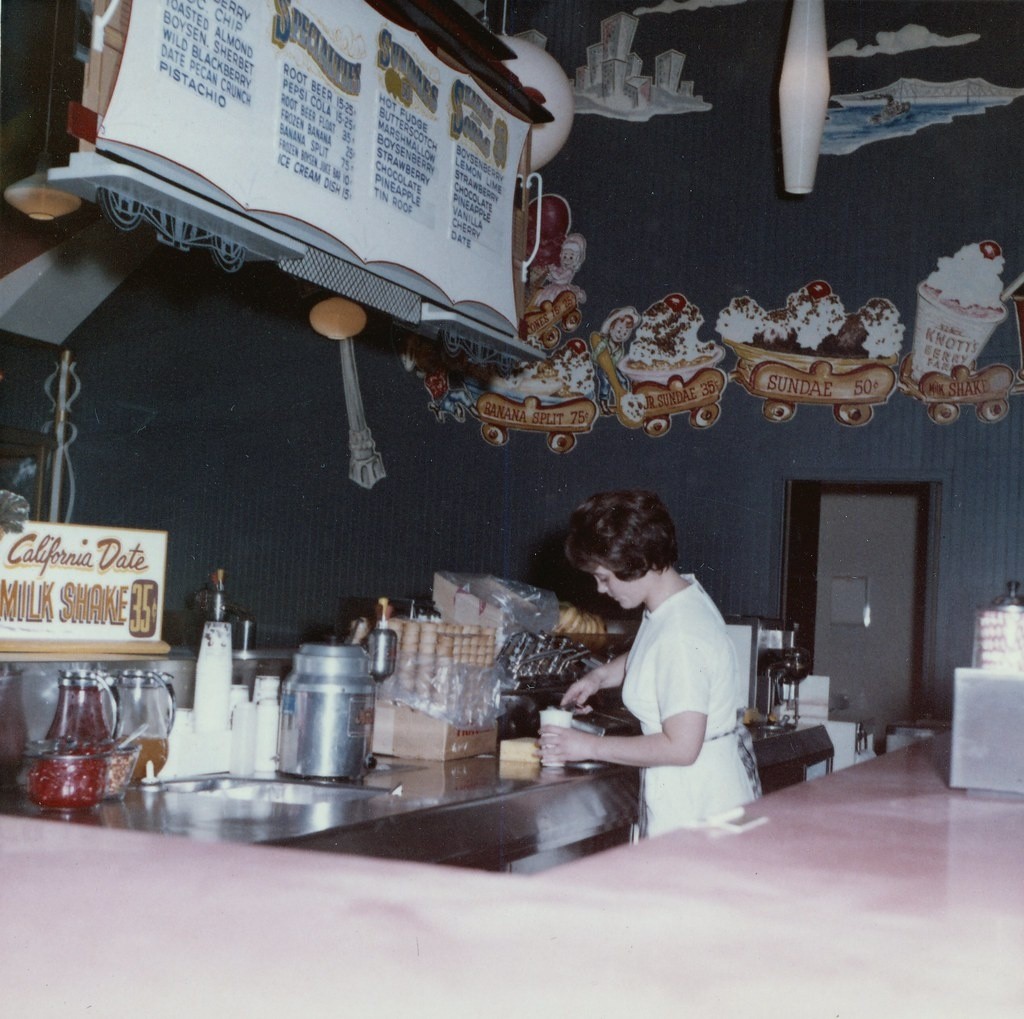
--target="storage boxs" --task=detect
[372,701,498,762]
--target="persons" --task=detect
[531,489,761,840]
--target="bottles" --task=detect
[277,639,376,780]
[46,669,117,745]
[1,663,29,764]
[112,670,174,781]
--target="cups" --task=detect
[539,711,572,767]
[147,621,281,780]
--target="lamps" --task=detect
[4,0,82,220]
[493,0,574,175]
[779,0,831,196]
[309,293,367,340]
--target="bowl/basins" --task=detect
[22,738,143,812]
